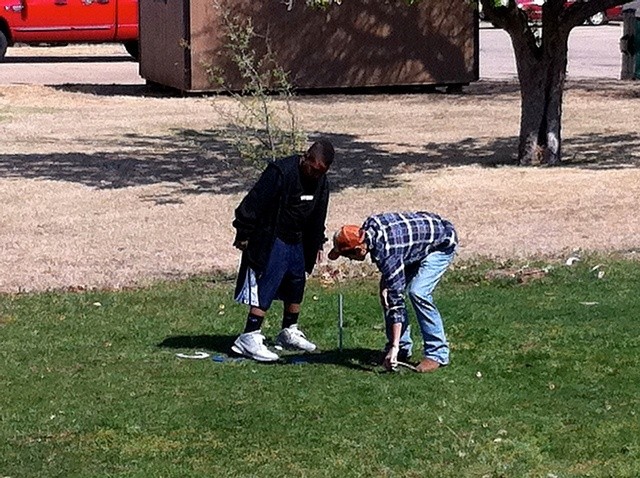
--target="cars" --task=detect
[0,0,141,60]
[514,0,623,26]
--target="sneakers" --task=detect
[230,330,279,361]
[416,358,441,372]
[276,328,316,352]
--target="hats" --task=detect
[329,225,366,260]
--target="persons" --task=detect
[327,211,459,374]
[233,139,335,363]
